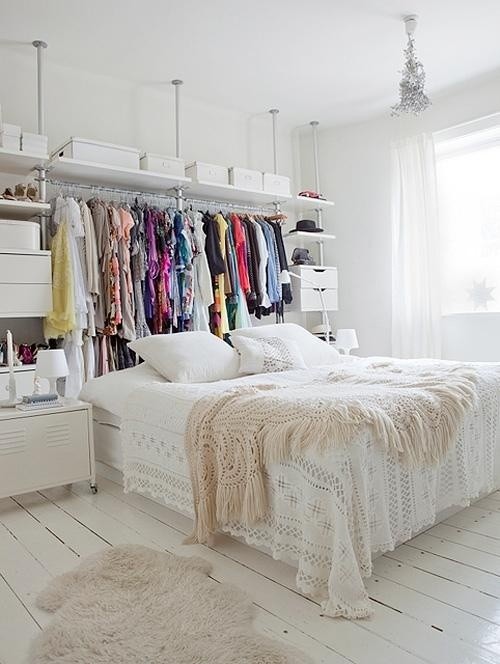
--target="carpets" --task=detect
[29,545,313,663]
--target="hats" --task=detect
[311,325,336,340]
[289,220,324,233]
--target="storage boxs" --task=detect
[0,124,48,158]
[51,137,290,196]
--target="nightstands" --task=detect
[0,397,97,499]
[0,364,36,402]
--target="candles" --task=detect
[6,330,14,373]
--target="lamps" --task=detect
[390,14,432,119]
[335,329,359,355]
[37,350,69,399]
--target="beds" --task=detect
[79,357,499,620]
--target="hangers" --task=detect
[48,174,280,233]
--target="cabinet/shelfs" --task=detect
[284,195,338,311]
[0,149,53,320]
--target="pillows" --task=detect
[126,332,253,383]
[228,335,308,373]
[224,324,340,366]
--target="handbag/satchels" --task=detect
[0,340,49,367]
[291,247,316,265]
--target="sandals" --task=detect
[0,180,45,203]
